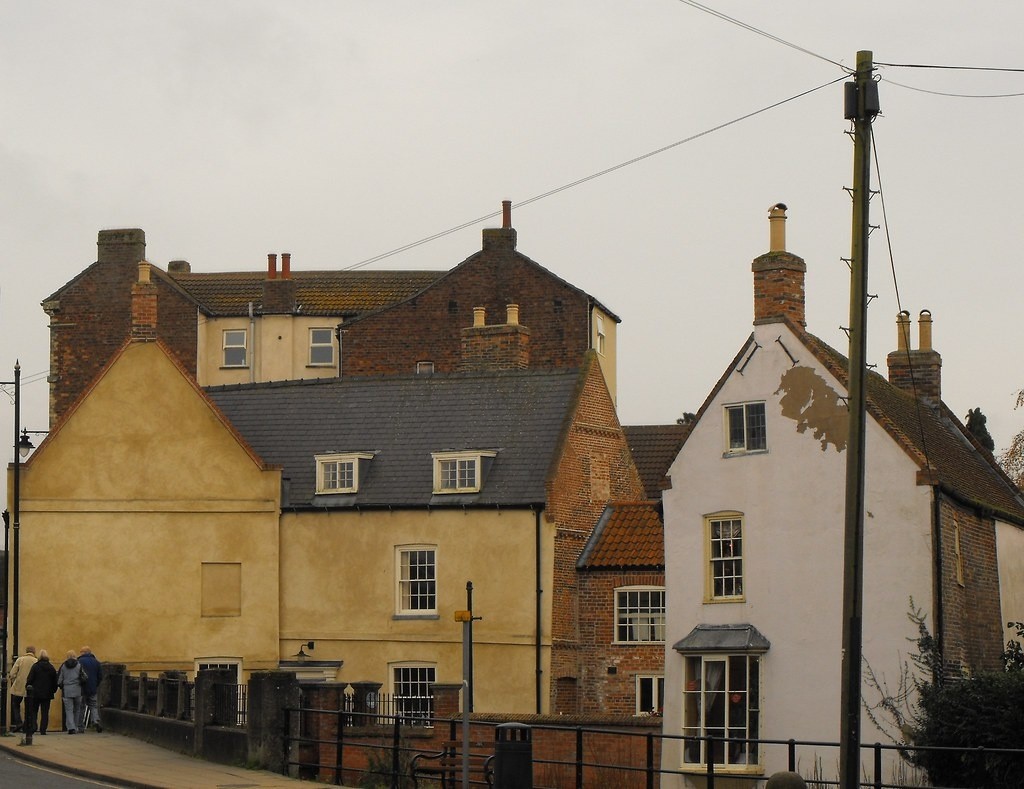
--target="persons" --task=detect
[9,645,103,746]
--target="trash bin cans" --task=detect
[350,679,383,726]
[494,722,533,789]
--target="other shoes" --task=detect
[68,729,75,734]
[12,724,24,733]
[94,721,104,733]
[40,729,46,735]
[77,728,84,733]
